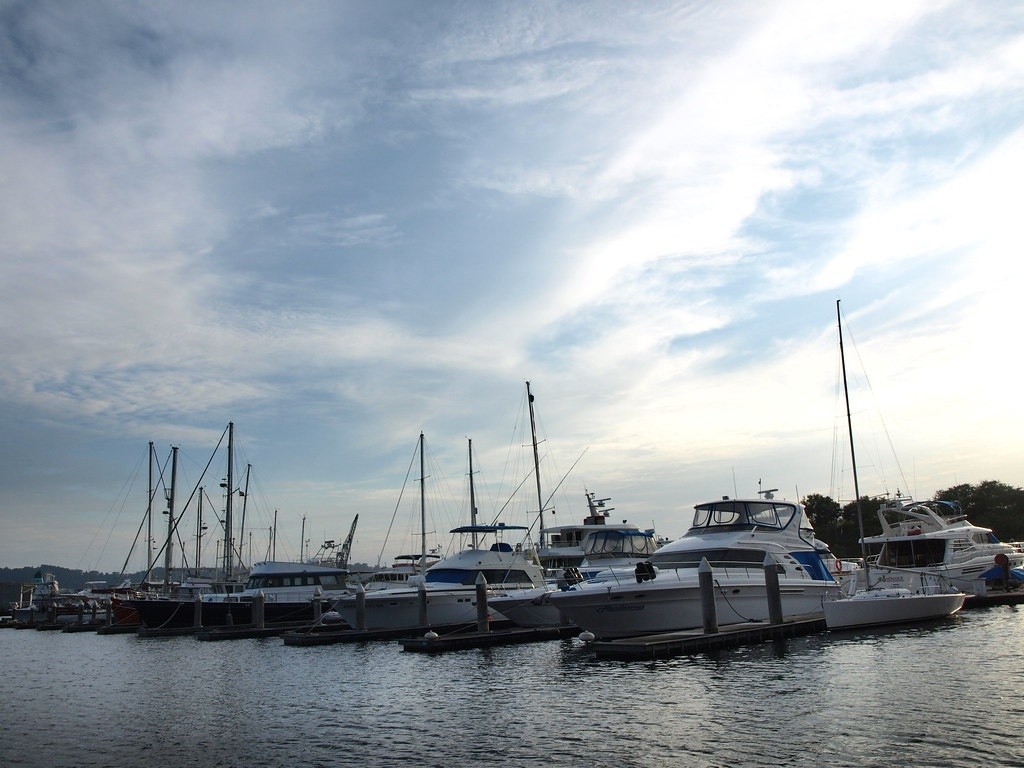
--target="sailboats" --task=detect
[819,300,970,629]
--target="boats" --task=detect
[828,491,1024,593]
[550,474,843,639]
[479,519,662,631]
[9,382,663,634]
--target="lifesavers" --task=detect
[836,560,843,570]
